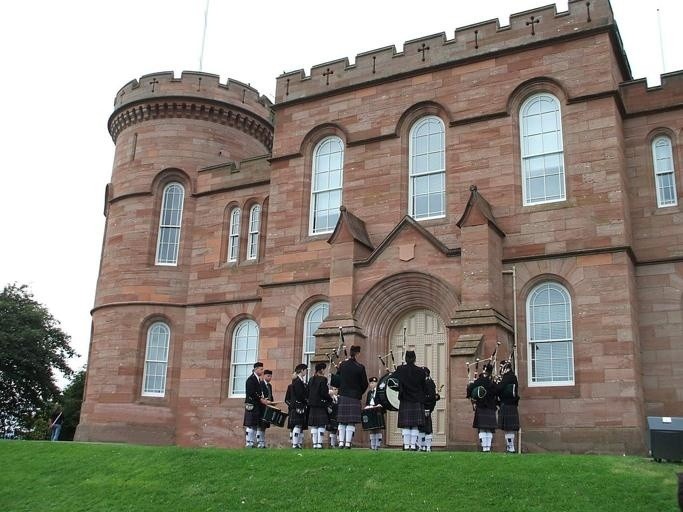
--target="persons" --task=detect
[284,363,340,449]
[466,363,498,452]
[391,350,440,451]
[48,404,64,441]
[497,360,520,453]
[331,345,369,449]
[255,369,274,448]
[367,376,387,450]
[243,362,271,449]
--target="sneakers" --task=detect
[241,442,516,454]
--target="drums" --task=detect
[261,405,281,424]
[361,407,385,430]
[274,411,288,427]
[377,372,401,411]
